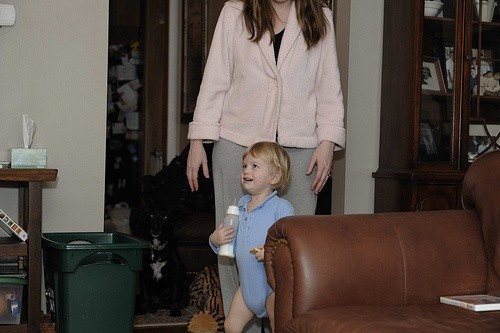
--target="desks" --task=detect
[0,169,58,333]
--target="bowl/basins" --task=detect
[424,1,444,17]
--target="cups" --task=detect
[473,1,496,22]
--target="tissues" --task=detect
[10,113,47,168]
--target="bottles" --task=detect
[217,197,239,257]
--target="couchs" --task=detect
[263,145,500,333]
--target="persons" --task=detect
[422,68,434,85]
[209,141,296,333]
[446,51,500,97]
[468,137,494,162]
[186,0,346,333]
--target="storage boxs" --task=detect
[0,277,28,325]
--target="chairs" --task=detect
[147,167,215,317]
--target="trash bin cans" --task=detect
[42,232,152,333]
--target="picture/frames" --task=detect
[180,0,208,125]
[468,120,500,165]
[421,56,448,95]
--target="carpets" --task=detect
[133,306,193,329]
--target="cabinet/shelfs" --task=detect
[372,0,500,213]
